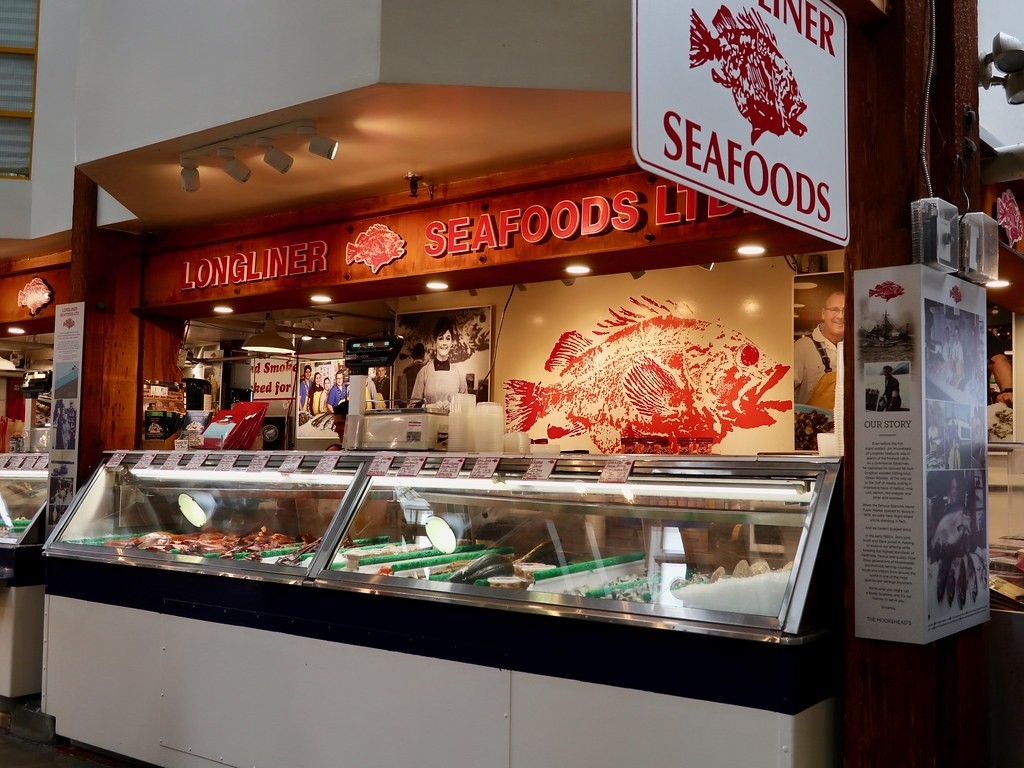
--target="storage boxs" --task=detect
[910,195,963,276]
[955,210,1000,286]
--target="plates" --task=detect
[299,410,311,427]
[794,403,833,419]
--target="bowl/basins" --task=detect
[446,393,561,455]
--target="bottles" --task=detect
[8,437,18,454]
[18,437,25,452]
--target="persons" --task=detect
[927,321,975,564]
[300,364,313,411]
[50,399,76,523]
[792,290,844,412]
[987,329,1014,408]
[374,366,391,409]
[307,372,323,416]
[319,375,331,414]
[402,343,428,403]
[326,370,347,414]
[406,317,469,406]
[877,366,902,411]
[365,375,380,411]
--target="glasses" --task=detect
[824,307,844,316]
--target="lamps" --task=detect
[296,127,339,160]
[214,148,251,185]
[180,158,200,194]
[241,312,297,356]
[256,136,294,176]
